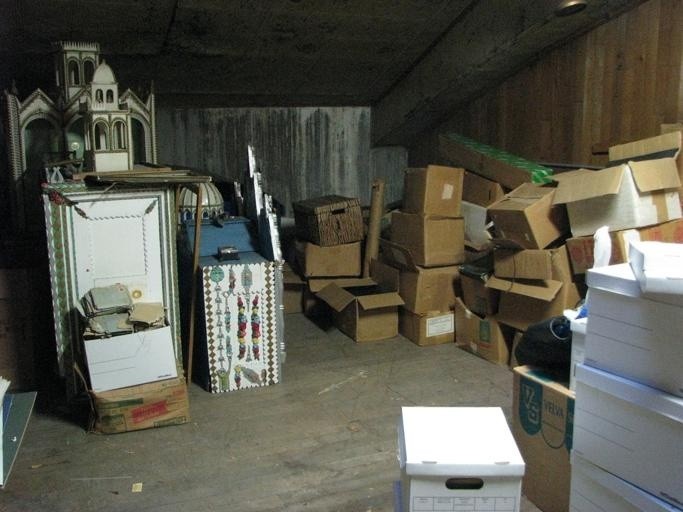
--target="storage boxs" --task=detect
[393,122,682,363]
[68,319,189,437]
[288,166,463,343]
[397,248,681,511]
